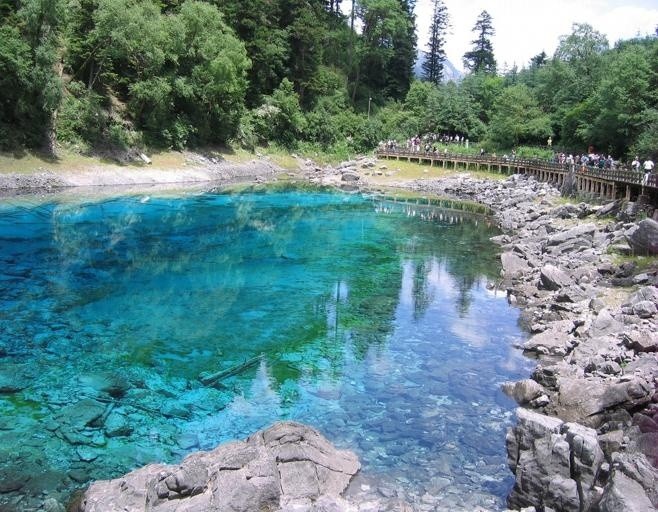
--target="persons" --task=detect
[379,132,469,157]
[479,145,654,182]
